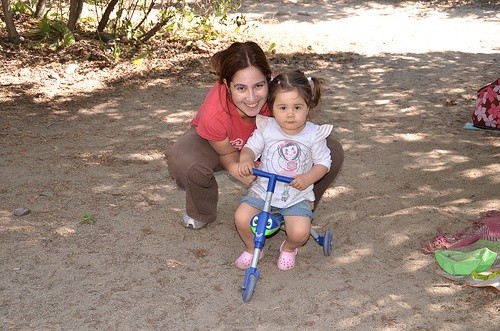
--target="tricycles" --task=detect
[242,167,332,301]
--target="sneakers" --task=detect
[182,213,208,229]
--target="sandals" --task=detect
[277,240,298,271]
[235,249,264,270]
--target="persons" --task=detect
[165,40,346,232]
[235,68,334,271]
